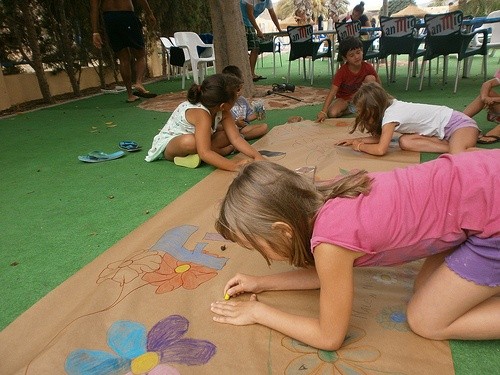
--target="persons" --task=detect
[341,1,372,39]
[240,0,286,79]
[462,68,500,144]
[222,65,268,140]
[210,149,500,351]
[314,36,379,123]
[90,0,157,102]
[334,81,488,156]
[371,18,376,35]
[145,73,265,172]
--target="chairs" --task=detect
[160,31,216,90]
[336,22,380,82]
[419,10,490,93]
[261,34,282,76]
[286,25,334,85]
[378,16,431,91]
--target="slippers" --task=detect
[126,96,140,103]
[133,90,158,98]
[253,76,267,81]
[478,132,500,144]
[77,150,125,162]
[118,141,139,151]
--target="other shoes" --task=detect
[174,154,200,168]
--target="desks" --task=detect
[361,17,500,83]
[260,31,336,88]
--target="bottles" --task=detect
[318,13,323,31]
[328,17,333,30]
